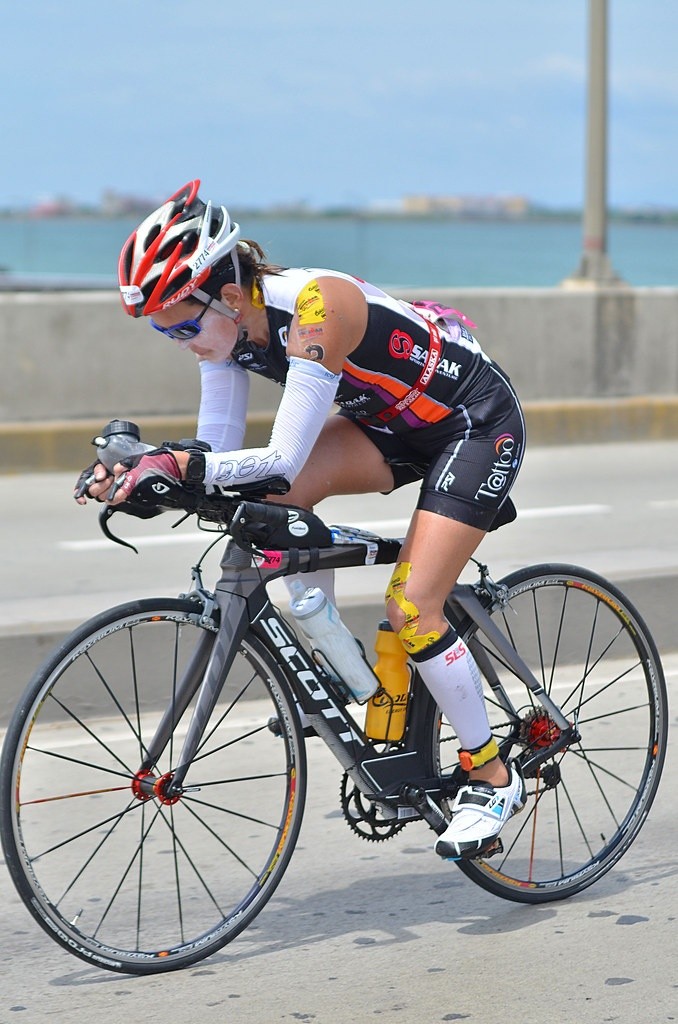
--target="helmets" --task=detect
[119,178,240,318]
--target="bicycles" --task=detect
[1,421,668,975]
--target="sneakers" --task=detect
[268,713,351,738]
[436,757,527,861]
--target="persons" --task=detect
[73,178,529,859]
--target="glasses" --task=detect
[151,290,219,339]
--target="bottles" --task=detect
[288,580,381,702]
[365,619,409,739]
